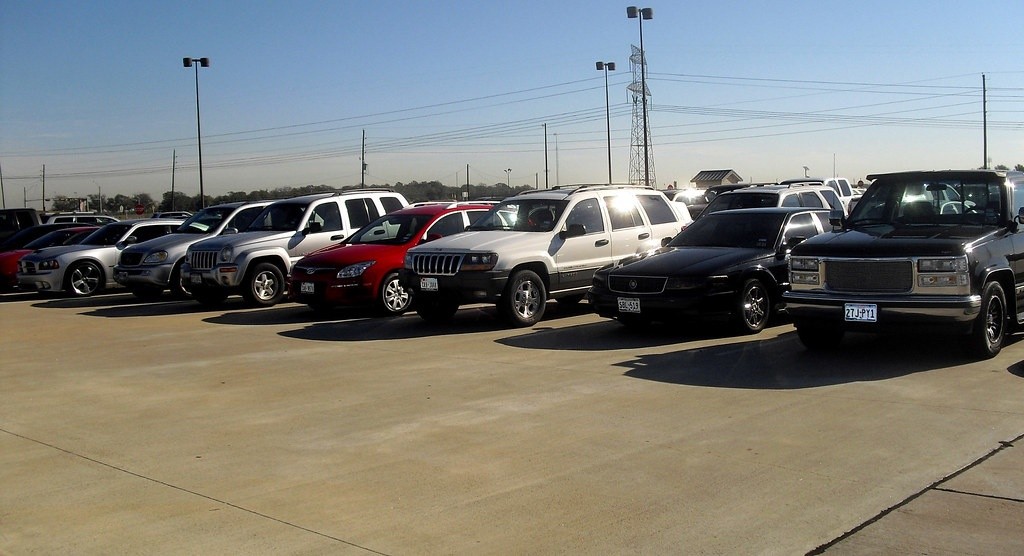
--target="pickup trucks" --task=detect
[846,183,978,220]
[780,177,865,210]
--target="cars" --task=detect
[899,200,978,217]
[0,227,100,285]
[151,211,193,219]
[958,184,1000,201]
[586,206,845,330]
[661,188,713,204]
[16,219,210,295]
[286,200,519,318]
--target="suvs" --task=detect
[399,182,684,326]
[36,211,120,227]
[0,208,44,252]
[781,167,1024,356]
[777,180,854,213]
[113,199,325,302]
[180,186,416,307]
[678,186,849,227]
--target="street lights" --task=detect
[183,57,209,210]
[504,169,512,187]
[595,60,615,184]
[627,6,653,185]
